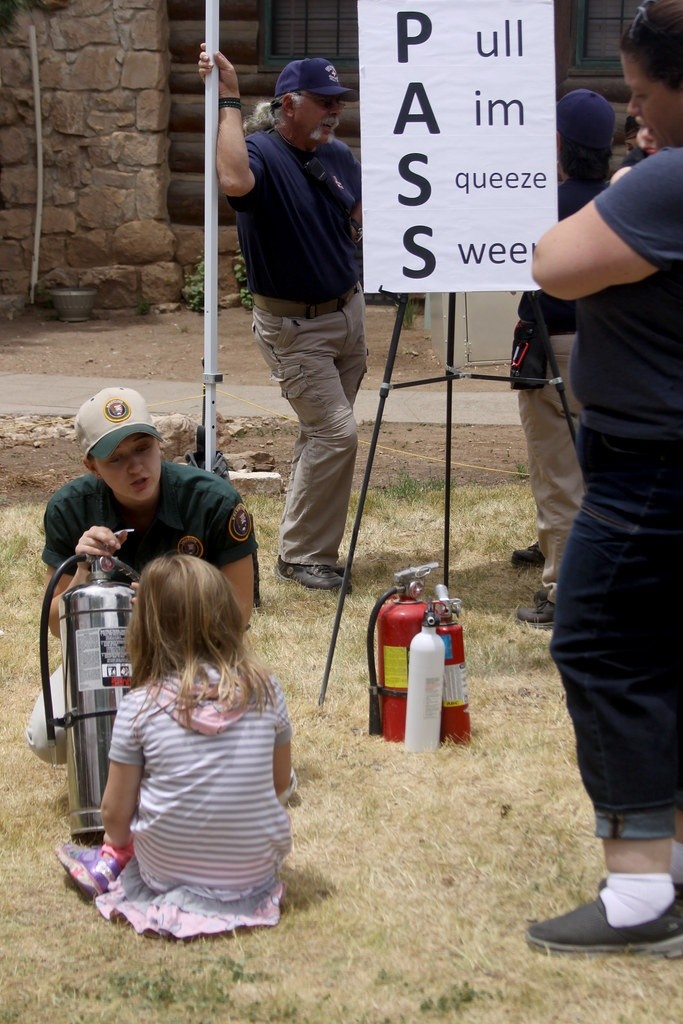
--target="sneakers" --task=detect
[518,601,560,629]
[55,841,121,900]
[272,555,351,594]
[510,540,546,567]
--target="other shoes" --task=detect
[528,896,683,958]
[599,877,683,901]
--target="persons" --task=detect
[610,115,655,187]
[530,0,683,955]
[52,550,294,938]
[24,386,259,764]
[198,42,368,593]
[509,89,615,629]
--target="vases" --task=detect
[51,287,98,322]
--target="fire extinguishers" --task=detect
[366,560,472,752]
[40,530,143,845]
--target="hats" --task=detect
[274,57,354,94]
[557,89,616,149]
[76,388,164,460]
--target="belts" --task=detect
[253,279,360,320]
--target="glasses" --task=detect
[631,0,677,51]
[297,92,345,107]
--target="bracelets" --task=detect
[219,97,241,111]
